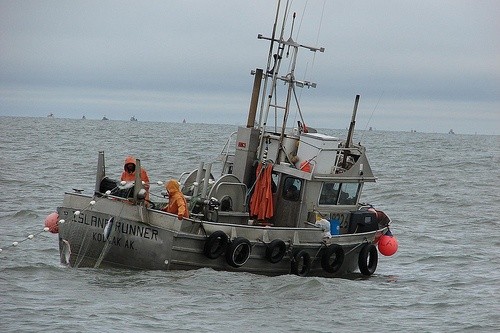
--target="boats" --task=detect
[59,0,390,275]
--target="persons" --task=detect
[122,157,151,207]
[159,178,189,220]
